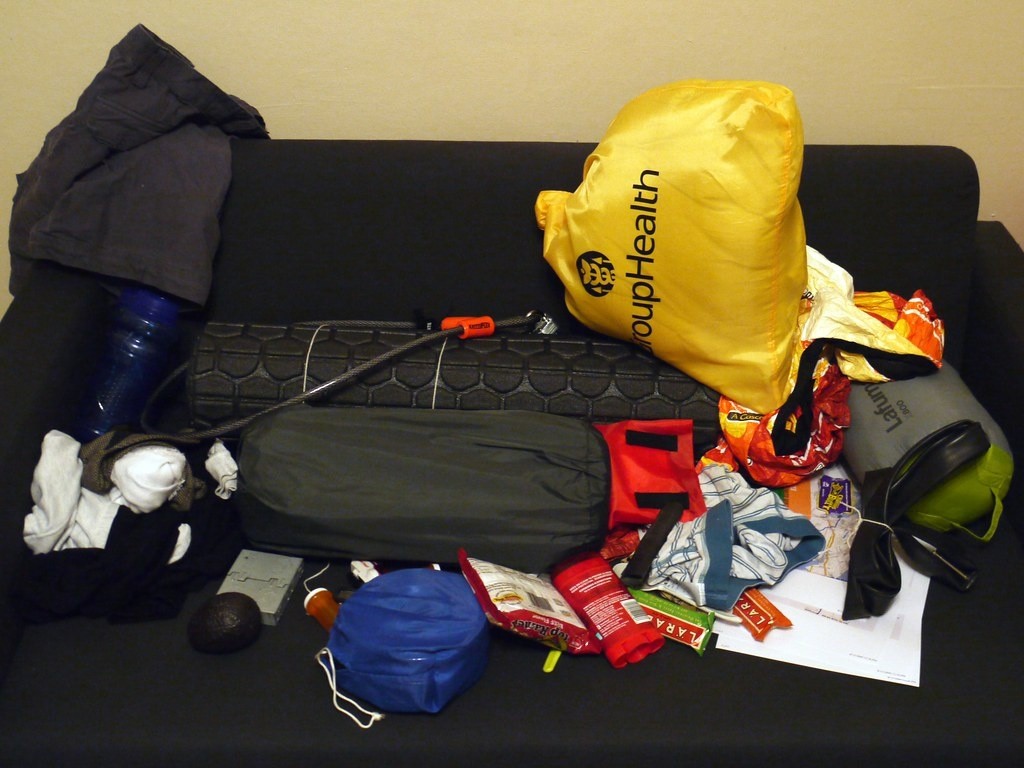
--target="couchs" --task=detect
[0,138,1024,768]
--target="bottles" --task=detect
[303,586,340,633]
[74,286,178,445]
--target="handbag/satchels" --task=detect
[311,567,488,728]
[535,77,807,414]
[216,404,611,571]
